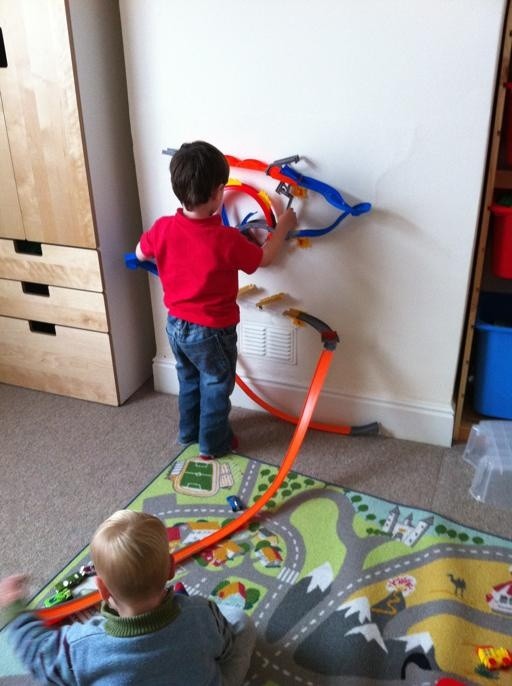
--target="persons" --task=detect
[1,509,257,686]
[134,140,296,460]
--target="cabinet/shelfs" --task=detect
[0,0,151,409]
